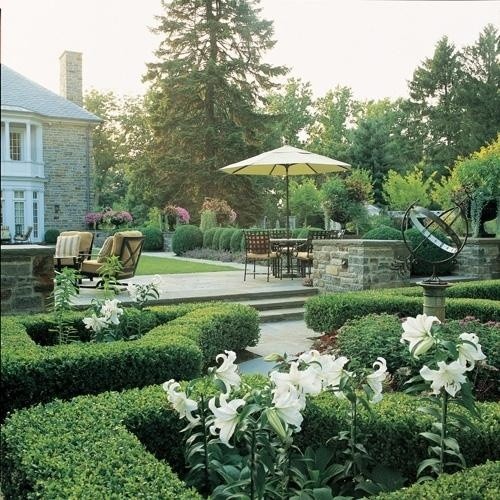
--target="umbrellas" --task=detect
[217,139,353,273]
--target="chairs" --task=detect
[240,226,347,283]
[52,229,148,297]
[1,223,34,245]
